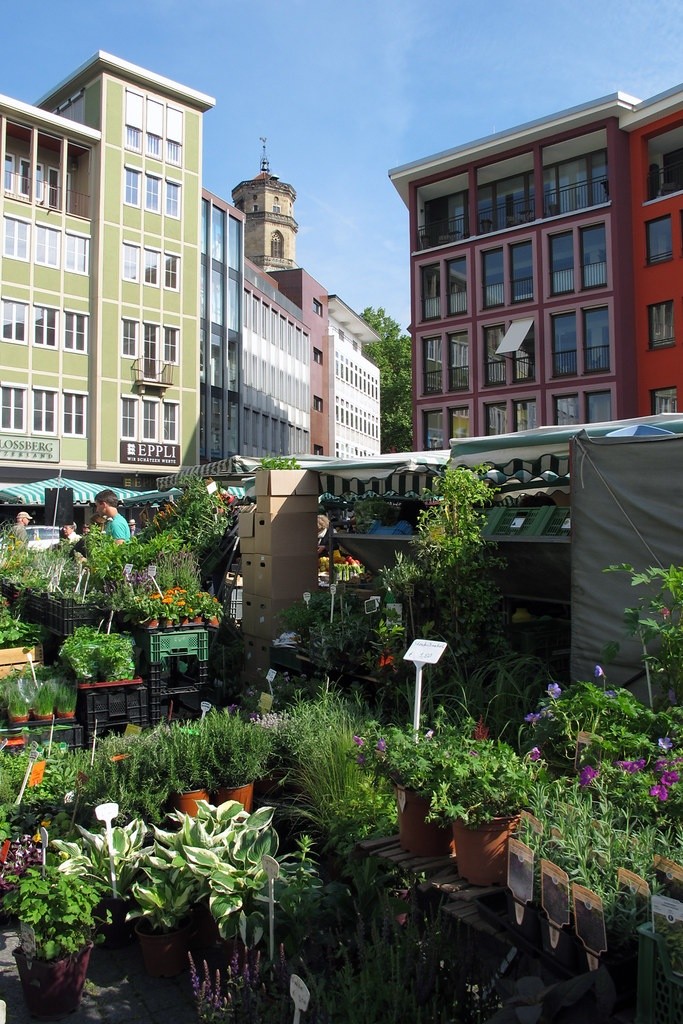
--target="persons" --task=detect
[94,489,130,545]
[64,521,82,545]
[317,516,339,558]
[6,512,33,549]
[138,506,149,530]
[83,524,91,536]
[128,519,141,537]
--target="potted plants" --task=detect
[57,682,76,718]
[538,853,596,963]
[23,691,36,720]
[309,614,353,670]
[208,705,267,814]
[32,686,54,721]
[505,818,549,940]
[165,723,210,817]
[61,822,146,941]
[8,690,30,723]
[126,830,195,978]
[573,873,638,998]
[480,219,493,232]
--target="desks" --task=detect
[519,209,533,222]
[448,231,461,242]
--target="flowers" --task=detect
[354,717,475,793]
[433,740,545,816]
[206,597,223,615]
[190,590,210,616]
[134,595,162,618]
[175,587,193,614]
[160,587,177,616]
[2,838,114,958]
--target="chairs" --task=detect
[545,204,559,218]
[439,233,447,244]
[506,217,517,227]
[421,235,434,249]
[124,724,143,739]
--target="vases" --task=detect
[194,615,202,624]
[142,619,158,627]
[395,789,451,855]
[451,811,519,885]
[208,617,220,627]
[180,616,190,624]
[11,939,94,1022]
[159,618,174,627]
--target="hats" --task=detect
[17,512,32,519]
[128,518,138,526]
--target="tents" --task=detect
[0,413,683,713]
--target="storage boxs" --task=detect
[255,514,317,557]
[46,590,103,638]
[255,554,318,599]
[255,637,269,677]
[255,596,295,640]
[487,506,547,536]
[534,506,572,537]
[142,628,209,662]
[242,592,255,637]
[367,520,413,536]
[238,513,257,554]
[473,507,499,536]
[242,553,255,595]
[245,635,256,675]
[2,580,16,605]
[255,469,319,513]
[26,587,47,625]
[77,685,149,735]
[139,661,208,695]
[503,619,571,660]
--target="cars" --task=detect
[0,522,81,553]
